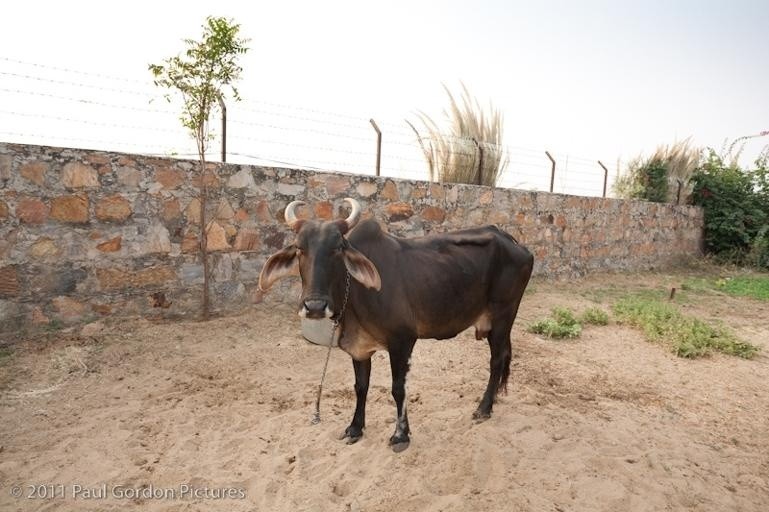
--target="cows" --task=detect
[258,196,535,453]
